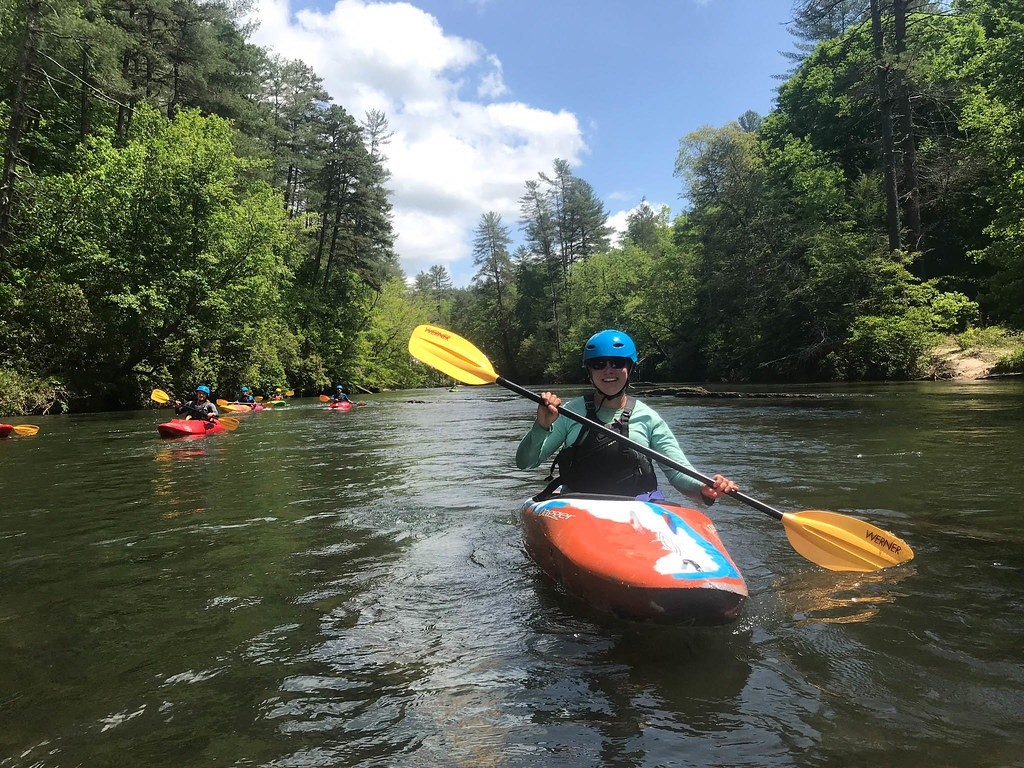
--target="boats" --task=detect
[219,402,262,413]
[518,476,749,627]
[267,400,287,408]
[158,418,227,438]
[329,402,352,411]
[449,388,458,392]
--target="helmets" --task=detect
[195,385,210,397]
[583,330,637,364]
[241,387,248,392]
[276,388,281,393]
[336,385,342,389]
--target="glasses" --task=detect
[586,356,630,370]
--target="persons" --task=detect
[451,383,457,389]
[269,388,284,401]
[331,385,353,403]
[235,386,255,406]
[175,386,219,421]
[515,330,739,507]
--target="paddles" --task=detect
[12,424,39,437]
[151,388,240,431]
[255,391,294,401]
[407,323,914,573]
[319,395,358,404]
[216,398,274,408]
